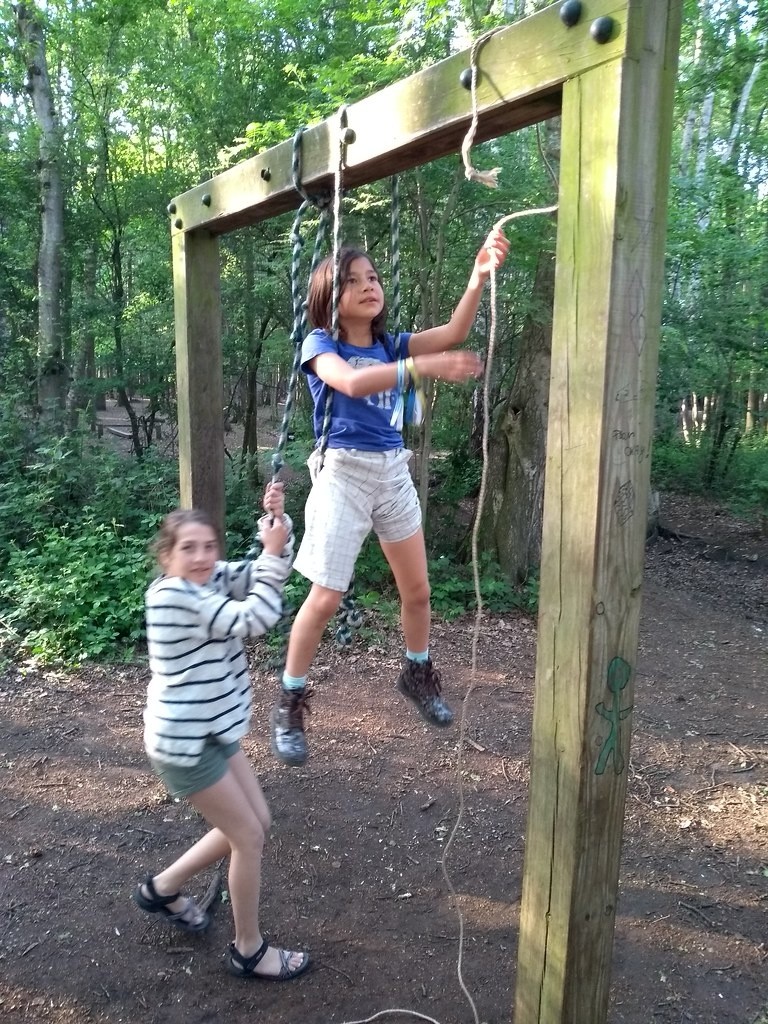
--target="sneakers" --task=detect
[270,676,316,766]
[396,656,454,728]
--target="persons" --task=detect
[131,478,310,980]
[272,225,512,766]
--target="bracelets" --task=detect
[397,356,421,396]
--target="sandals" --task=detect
[134,875,209,932]
[229,937,309,980]
